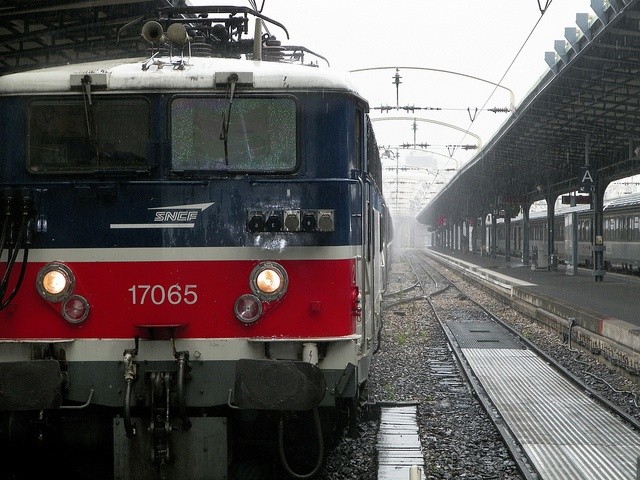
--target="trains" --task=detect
[0,10,392,478]
[491,196,640,277]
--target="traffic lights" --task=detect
[440,216,444,224]
